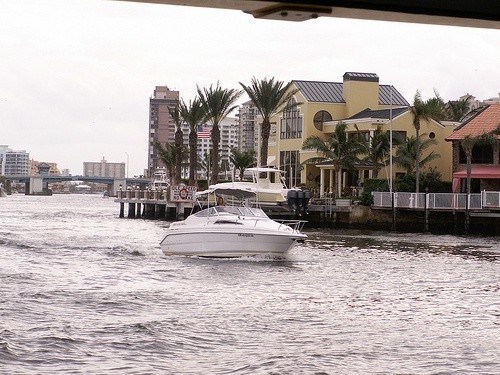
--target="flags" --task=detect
[196,125,214,139]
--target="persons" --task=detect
[216,196,225,206]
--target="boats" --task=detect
[159,188,309,258]
